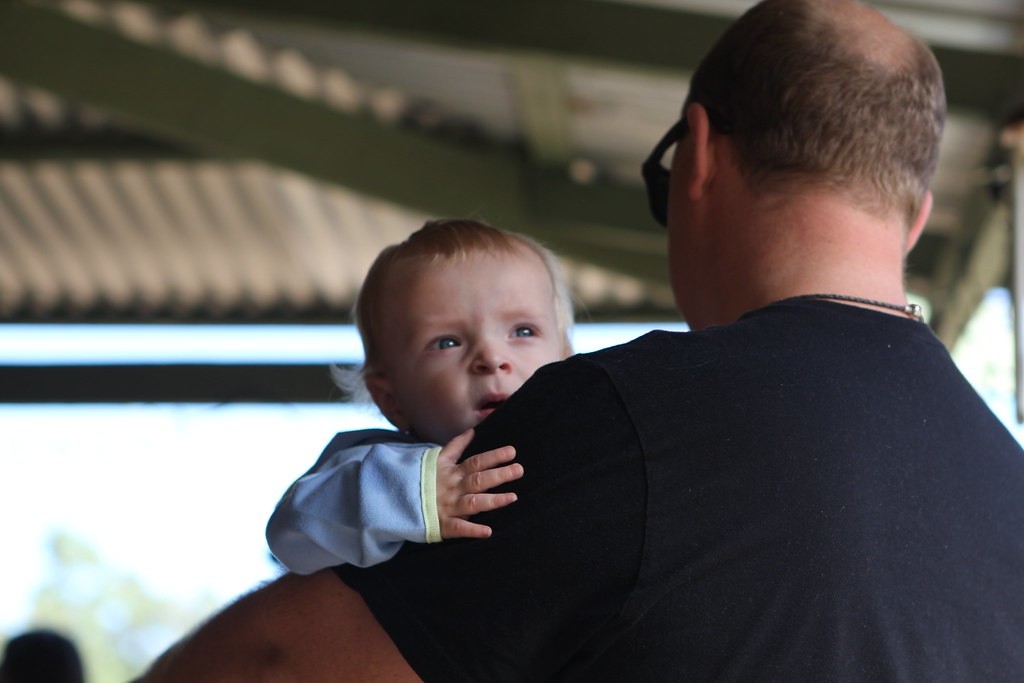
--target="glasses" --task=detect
[642,115,731,227]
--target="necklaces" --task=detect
[778,293,925,322]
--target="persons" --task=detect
[0,631,84,683]
[118,0,1024,683]
[266,218,574,578]
